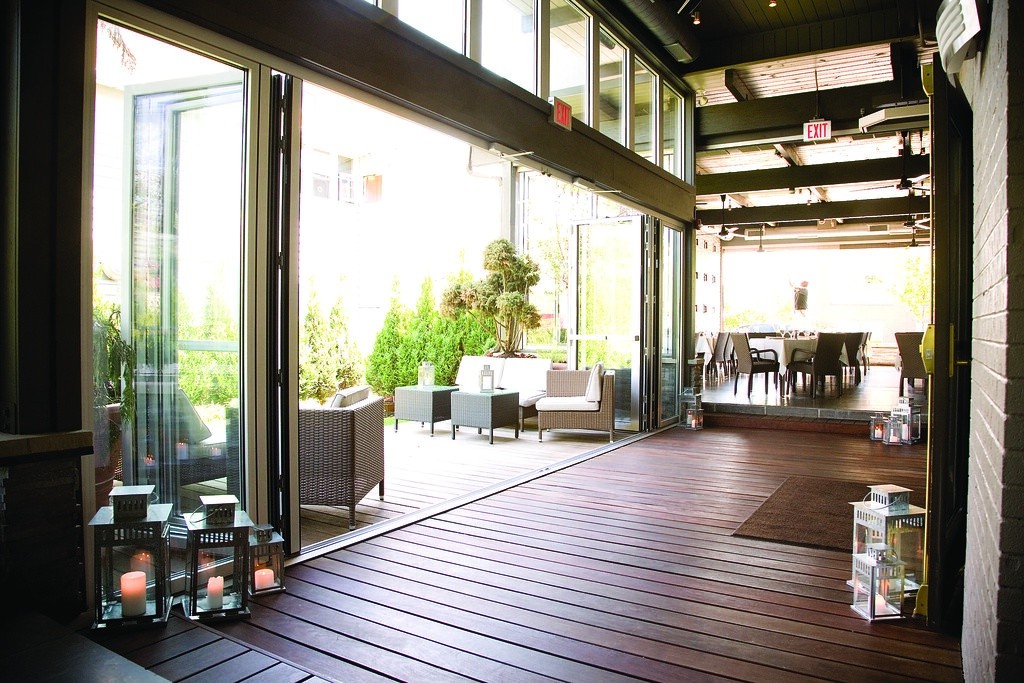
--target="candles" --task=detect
[867,591,885,614]
[875,426,883,437]
[120,569,146,613]
[890,428,898,442]
[902,424,912,440]
[692,420,696,428]
[878,552,898,590]
[687,410,693,424]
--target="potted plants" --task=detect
[88,306,139,506]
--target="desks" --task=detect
[451,390,520,444]
[691,330,723,383]
[394,384,460,437]
[744,333,870,398]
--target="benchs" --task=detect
[454,355,566,432]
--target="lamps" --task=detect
[908,215,918,246]
[719,194,728,237]
[905,192,918,228]
[754,224,766,255]
[898,133,913,190]
[255,566,273,588]
[935,0,986,87]
[207,574,224,607]
[692,12,700,25]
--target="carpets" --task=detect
[732,475,880,552]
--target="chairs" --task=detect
[225,383,386,530]
[150,388,229,491]
[536,362,615,444]
[694,328,932,401]
